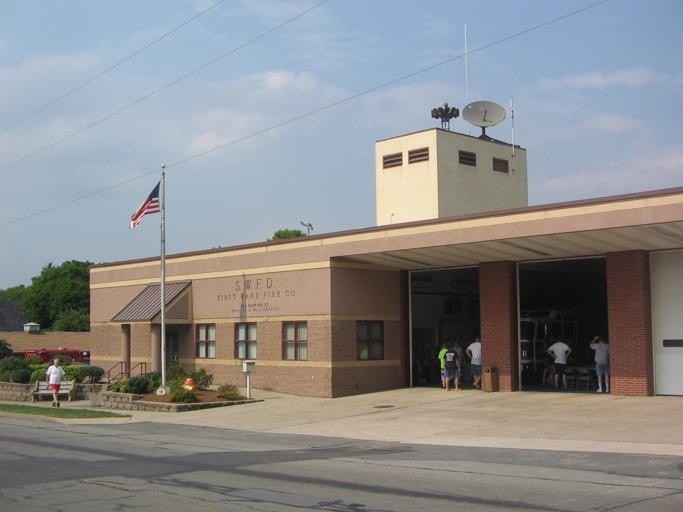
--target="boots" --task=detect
[597,383,609,392]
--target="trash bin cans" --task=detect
[481,366,498,392]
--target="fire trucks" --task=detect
[520,309,608,386]
[23,347,90,365]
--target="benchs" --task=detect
[31,381,77,402]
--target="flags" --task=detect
[129,179,162,229]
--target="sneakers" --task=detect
[52,402,60,407]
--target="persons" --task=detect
[437,342,450,389]
[546,336,572,389]
[450,340,466,384]
[45,358,66,408]
[589,336,610,393]
[443,345,460,391]
[464,336,482,388]
